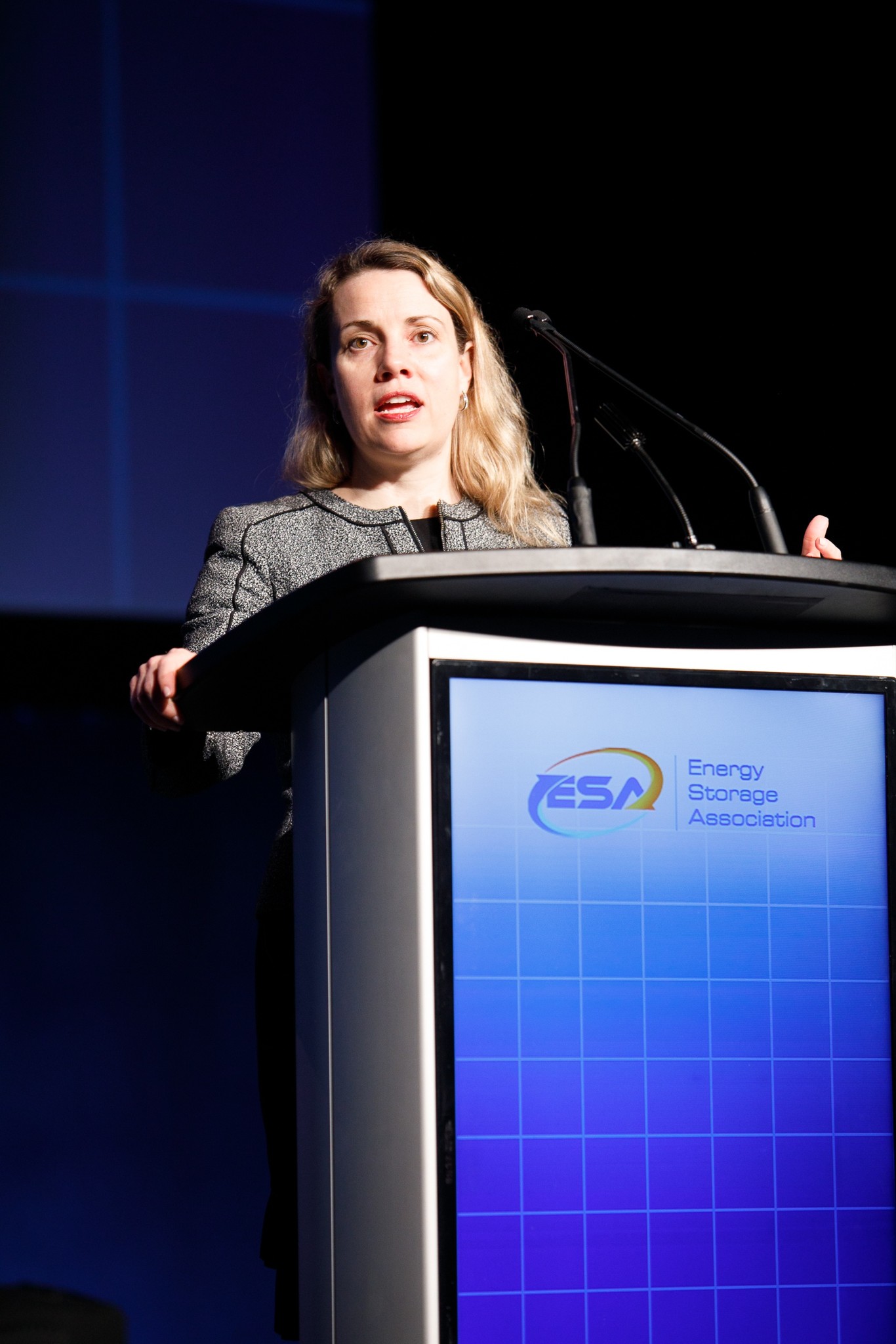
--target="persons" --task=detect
[129,240,843,1344]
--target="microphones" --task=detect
[512,299,798,557]
[594,398,719,550]
